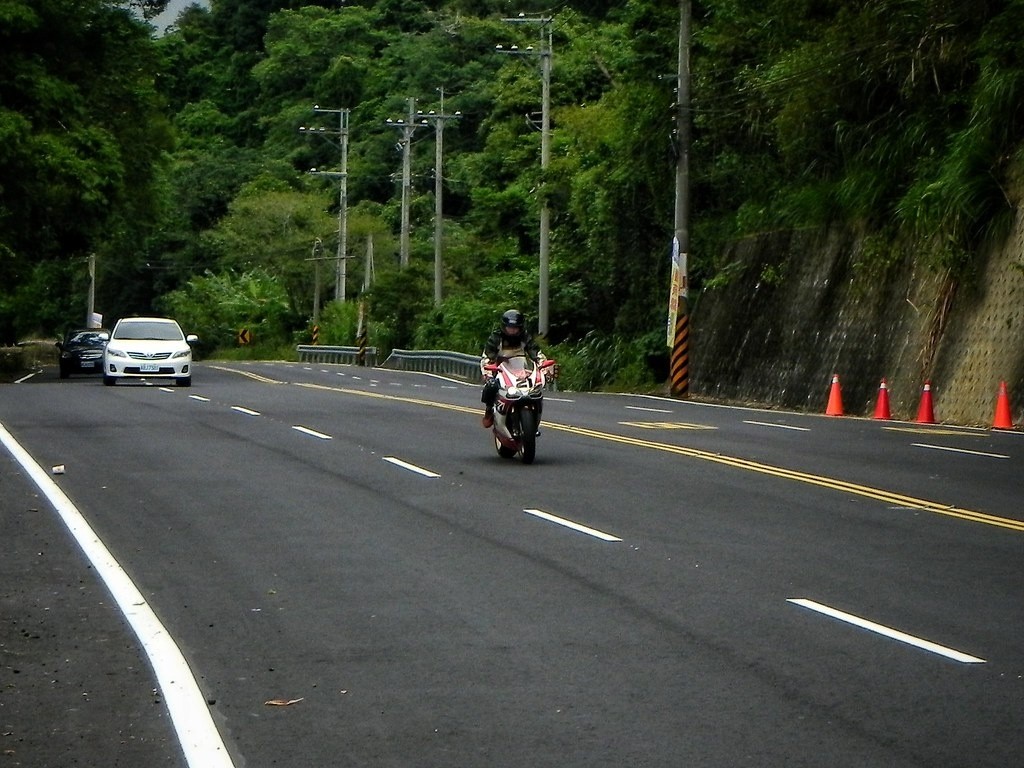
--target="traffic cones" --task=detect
[870,378,894,421]
[827,374,844,416]
[992,379,1018,431]
[914,379,937,424]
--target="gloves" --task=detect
[483,377,500,393]
[545,363,562,384]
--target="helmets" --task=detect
[501,308,525,340]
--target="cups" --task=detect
[52,465,64,473]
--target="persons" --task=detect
[481,309,547,427]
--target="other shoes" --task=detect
[483,408,495,428]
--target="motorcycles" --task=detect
[480,352,556,464]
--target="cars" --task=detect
[97,317,200,387]
[53,327,110,380]
